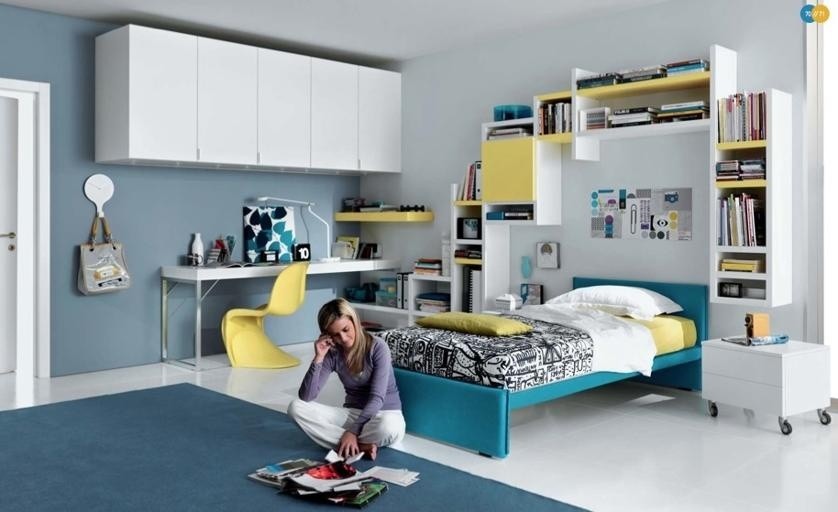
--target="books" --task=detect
[411,255,449,313]
[330,234,383,261]
[359,203,398,212]
[721,335,788,347]
[248,448,423,507]
[716,91,768,297]
[533,54,708,140]
[448,101,533,263]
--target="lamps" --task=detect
[257,195,339,262]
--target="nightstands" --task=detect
[700,335,832,436]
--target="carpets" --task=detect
[0,381,585,510]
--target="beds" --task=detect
[353,278,709,460]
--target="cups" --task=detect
[185,253,203,266]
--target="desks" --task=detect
[160,258,400,371]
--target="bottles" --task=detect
[191,232,203,266]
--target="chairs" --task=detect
[218,261,310,368]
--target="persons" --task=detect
[288,296,407,462]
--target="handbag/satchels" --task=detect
[77,215,131,297]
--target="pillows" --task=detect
[414,311,534,338]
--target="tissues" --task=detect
[496,293,523,311]
